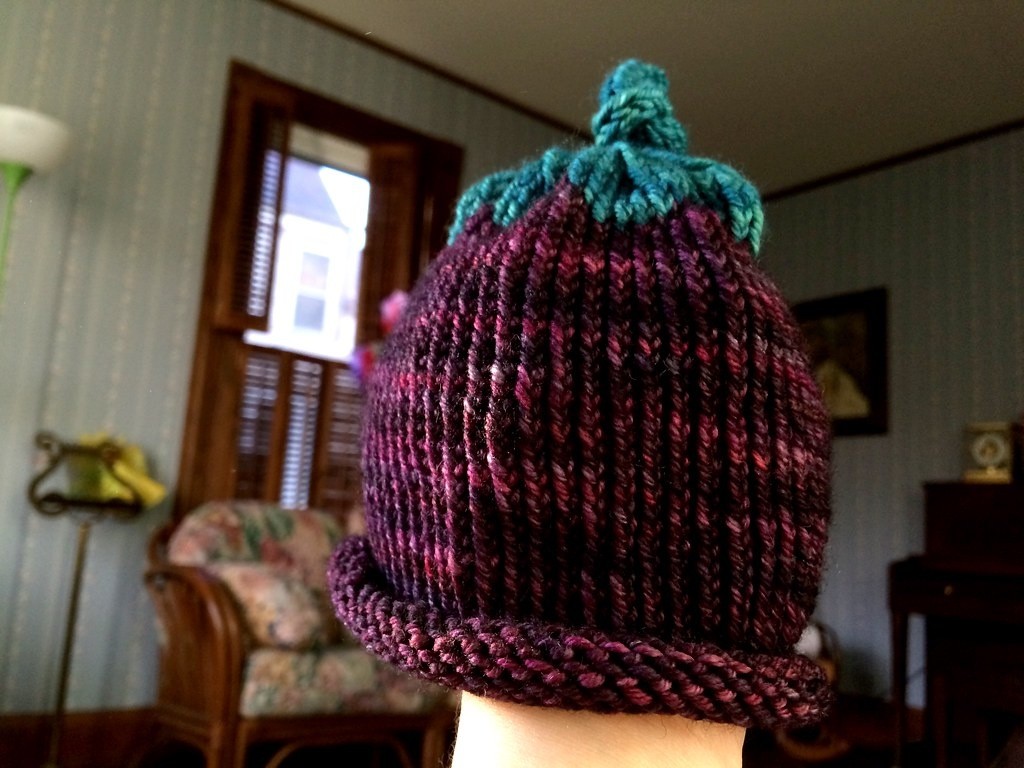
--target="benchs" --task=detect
[140,495,466,768]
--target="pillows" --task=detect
[204,560,336,651]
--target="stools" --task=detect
[929,645,1024,768]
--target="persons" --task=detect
[325,56,834,768]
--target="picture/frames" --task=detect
[788,286,889,438]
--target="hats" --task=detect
[328,59,835,729]
